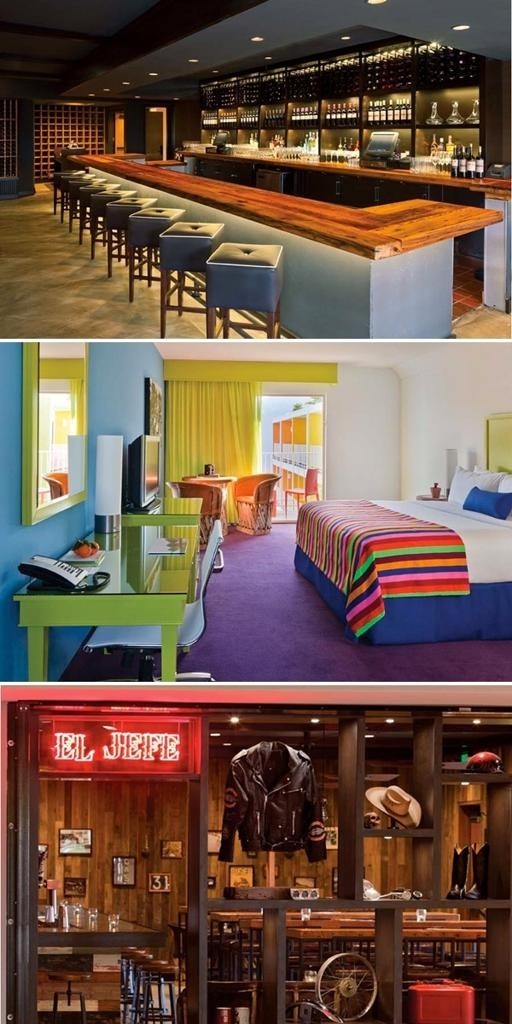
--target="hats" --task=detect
[365,785,422,829]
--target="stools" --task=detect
[47,158,287,339]
[121,941,177,1024]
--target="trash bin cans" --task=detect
[409,984,475,1024]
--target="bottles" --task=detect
[367,99,413,126]
[467,99,479,125]
[446,101,465,126]
[431,133,456,154]
[264,110,287,129]
[450,143,485,180]
[424,100,444,126]
[326,104,358,127]
[317,153,345,162]
[250,131,358,152]
[43,889,68,930]
[203,40,478,108]
[291,106,318,127]
[430,483,442,498]
[202,111,258,129]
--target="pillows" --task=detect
[460,485,512,521]
[446,468,500,505]
[497,474,512,492]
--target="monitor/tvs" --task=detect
[212,131,229,145]
[364,132,398,156]
[128,434,165,512]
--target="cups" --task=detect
[304,970,317,982]
[415,909,428,922]
[204,464,215,476]
[69,902,119,929]
[409,156,432,175]
[299,908,312,922]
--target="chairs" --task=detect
[284,467,321,519]
[158,494,203,515]
[167,481,229,551]
[83,514,232,681]
[41,471,66,497]
[233,472,281,536]
[176,905,486,981]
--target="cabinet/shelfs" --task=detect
[343,174,379,208]
[380,180,423,205]
[272,403,322,506]
[187,706,512,1024]
[445,188,484,263]
[199,33,503,165]
[296,166,341,202]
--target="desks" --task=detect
[16,514,202,681]
[182,474,237,535]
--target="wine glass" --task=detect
[432,154,450,177]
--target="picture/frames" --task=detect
[323,826,341,849]
[291,872,317,888]
[146,871,173,893]
[226,862,254,886]
[109,853,139,888]
[206,828,223,855]
[63,875,86,900]
[58,825,95,856]
[159,839,184,858]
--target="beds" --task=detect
[291,411,512,644]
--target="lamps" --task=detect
[441,448,459,497]
[95,433,125,535]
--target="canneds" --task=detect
[234,1007,250,1024]
[216,1007,231,1024]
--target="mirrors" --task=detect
[20,340,91,526]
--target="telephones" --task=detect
[18,555,89,590]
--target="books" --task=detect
[59,549,106,566]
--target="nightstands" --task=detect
[415,492,445,501]
